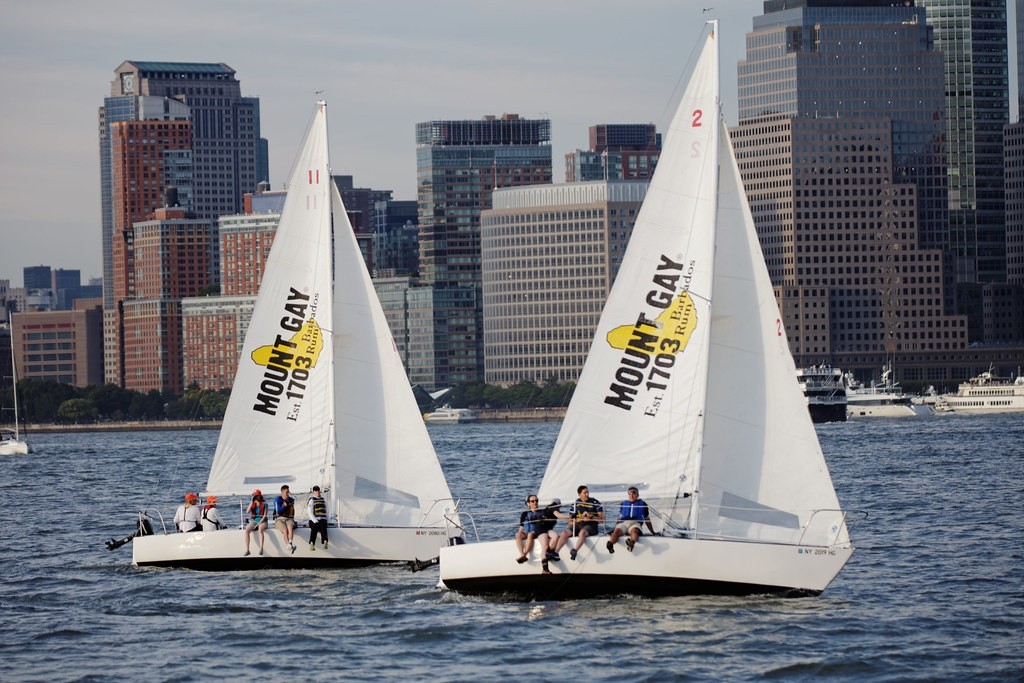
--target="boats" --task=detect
[795,366,1024,422]
[424,403,477,424]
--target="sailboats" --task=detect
[0,309,29,453]
[105,101,466,569]
[437,18,858,598]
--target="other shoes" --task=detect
[259,550,264,555]
[310,544,316,552]
[244,551,251,556]
[290,546,297,554]
[324,542,329,550]
[607,541,615,554]
[542,570,552,575]
[570,548,577,560]
[626,538,634,552]
[546,550,561,561]
[286,544,293,549]
[516,556,529,564]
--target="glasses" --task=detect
[529,500,538,503]
[628,490,637,495]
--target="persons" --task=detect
[545,485,604,561]
[517,498,570,564]
[515,495,553,574]
[307,486,328,551]
[606,487,664,554]
[273,485,296,554]
[200,496,227,532]
[243,490,268,556]
[174,493,203,532]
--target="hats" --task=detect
[253,490,262,497]
[207,496,219,502]
[185,494,198,501]
[312,485,320,491]
